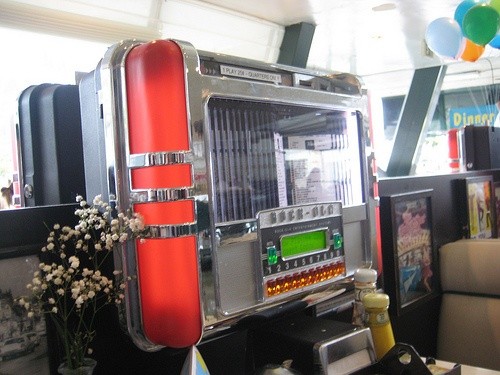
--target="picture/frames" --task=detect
[457,175,500,239]
[381,188,442,317]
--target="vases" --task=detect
[58,358,97,375]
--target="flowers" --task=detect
[13,195,145,368]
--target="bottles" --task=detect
[364,293,395,360]
[353,269,377,330]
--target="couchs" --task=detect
[438,240,500,372]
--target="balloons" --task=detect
[425,0,500,64]
